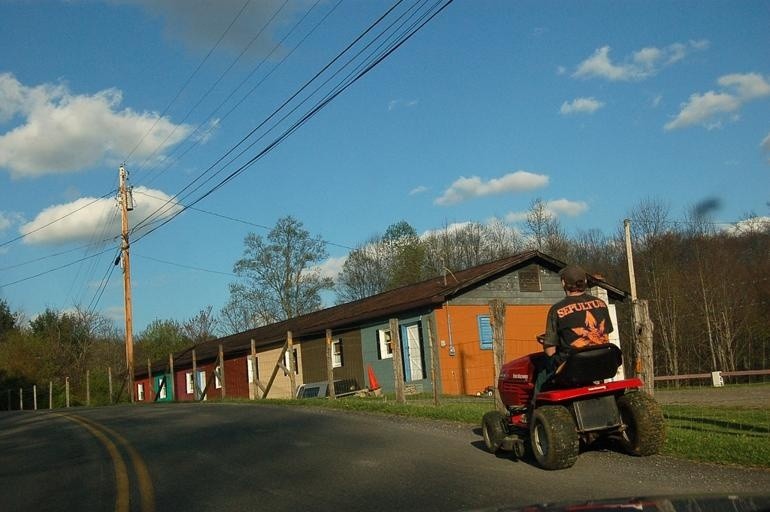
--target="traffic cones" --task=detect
[366,365,382,392]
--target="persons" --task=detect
[519,265,614,423]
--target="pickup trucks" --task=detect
[296,377,383,399]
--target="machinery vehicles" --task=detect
[479,333,662,470]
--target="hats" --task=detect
[558,266,585,286]
